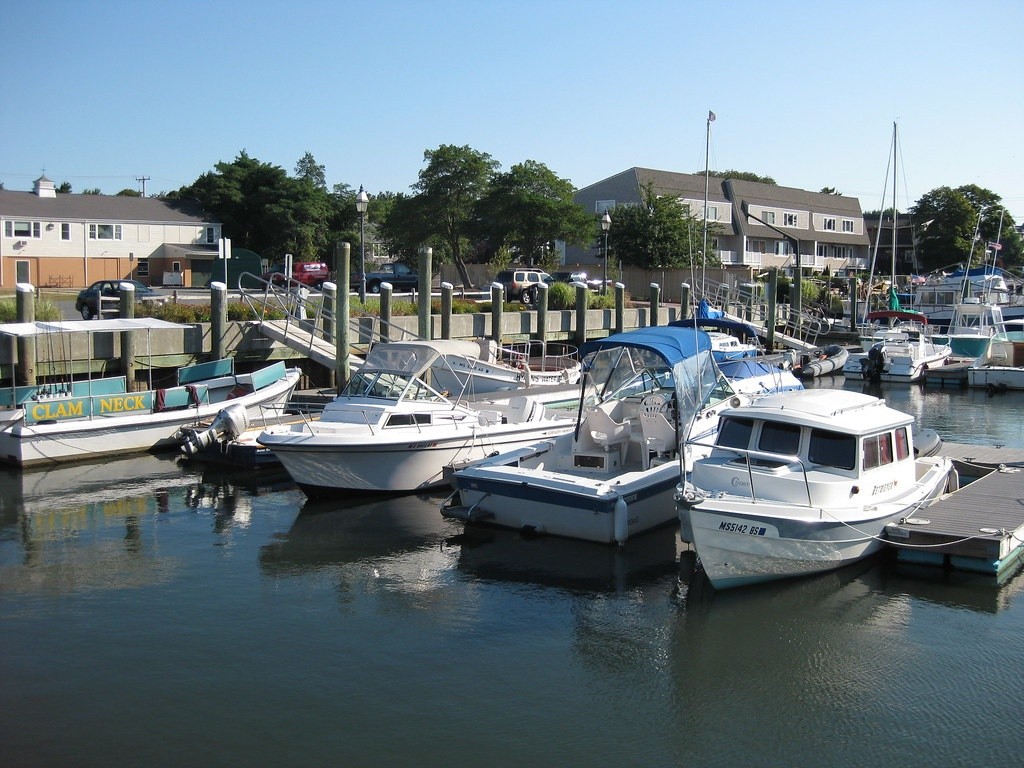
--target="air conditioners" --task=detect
[19,240,27,246]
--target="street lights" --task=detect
[354,184,370,305]
[600,208,613,296]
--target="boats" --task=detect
[176,401,324,468]
[0,354,308,472]
[257,332,612,505]
[668,326,959,597]
[177,119,1024,394]
[436,294,805,551]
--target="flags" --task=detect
[991,242,1001,250]
[911,274,925,283]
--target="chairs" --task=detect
[584,405,631,466]
[639,410,681,468]
[618,394,668,466]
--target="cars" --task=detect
[352,261,418,295]
[75,279,172,320]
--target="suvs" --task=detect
[495,267,556,305]
[550,270,588,285]
[260,258,328,292]
